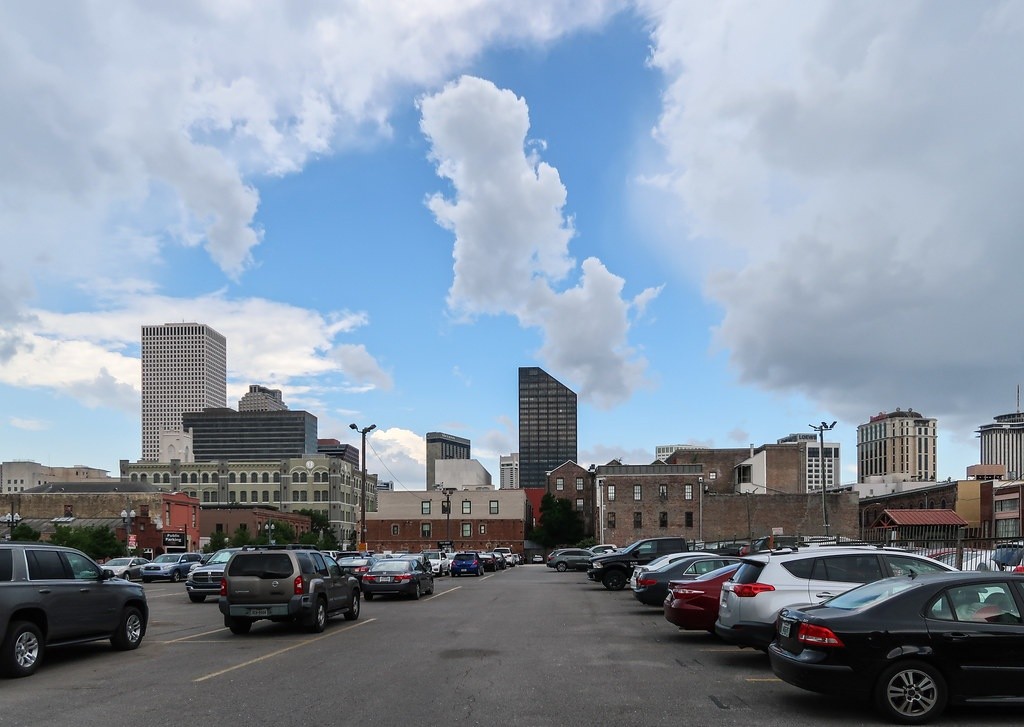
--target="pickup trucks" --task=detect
[585,537,740,592]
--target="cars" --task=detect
[189,550,528,591]
[99,557,151,581]
[361,557,434,601]
[768,570,1024,726]
[451,552,485,577]
[532,554,544,564]
[588,533,1023,660]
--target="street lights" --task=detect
[349,423,377,543]
[861,502,881,540]
[5,501,22,541]
[442,489,453,541]
[808,420,838,538]
[120,500,136,557]
[265,517,276,545]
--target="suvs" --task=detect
[0,540,149,680]
[492,546,516,567]
[140,551,203,583]
[185,546,262,603]
[714,546,1013,654]
[219,542,361,634]
[546,548,598,572]
